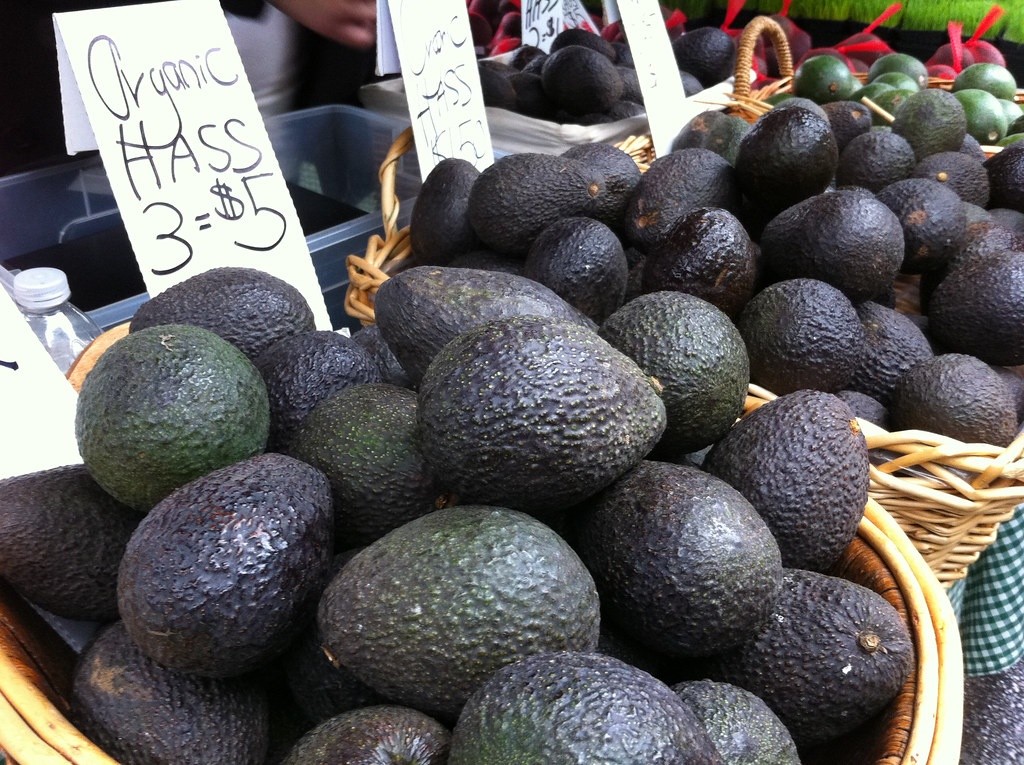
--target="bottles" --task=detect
[13,267,104,376]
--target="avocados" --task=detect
[1,23,1024,765]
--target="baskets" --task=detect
[0,15,1024,765]
